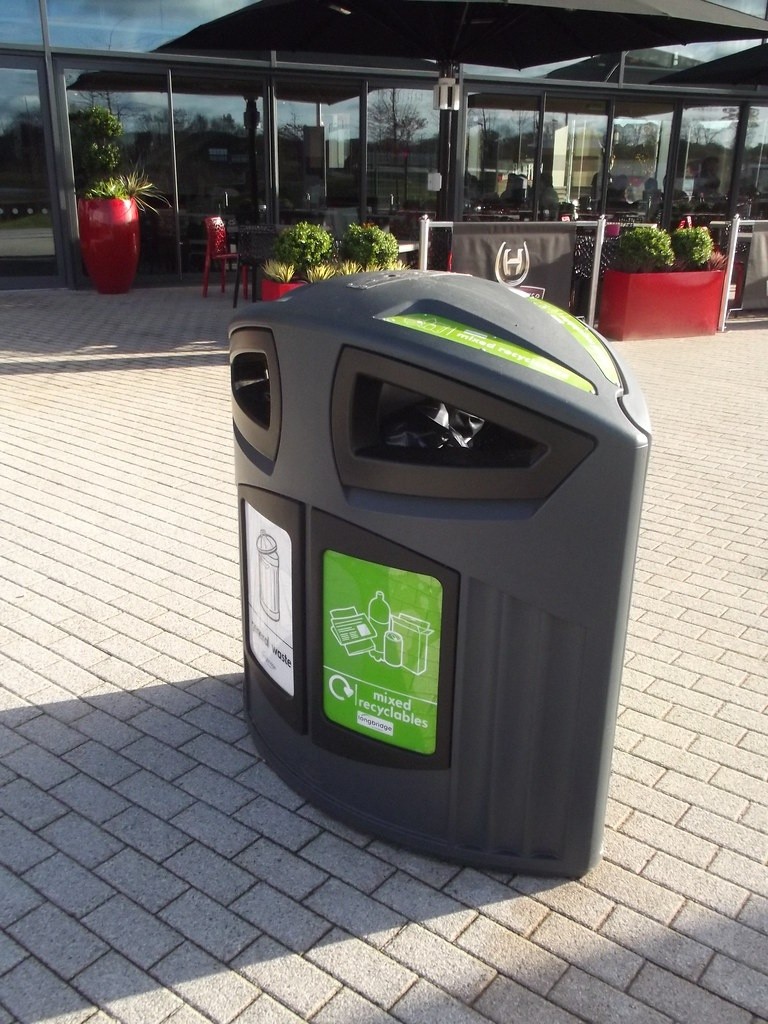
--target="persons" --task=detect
[591,154,614,198]
[541,173,558,207]
[693,157,721,193]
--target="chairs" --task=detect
[233,227,280,307]
[202,216,249,300]
[688,215,744,318]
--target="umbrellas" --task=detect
[149,0,768,220]
[65,0,438,221]
[548,48,702,118]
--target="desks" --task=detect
[710,220,768,243]
[576,221,657,230]
[336,241,431,266]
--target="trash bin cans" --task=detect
[223,271,657,885]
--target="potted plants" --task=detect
[74,105,173,294]
[261,222,338,302]
[599,226,727,342]
